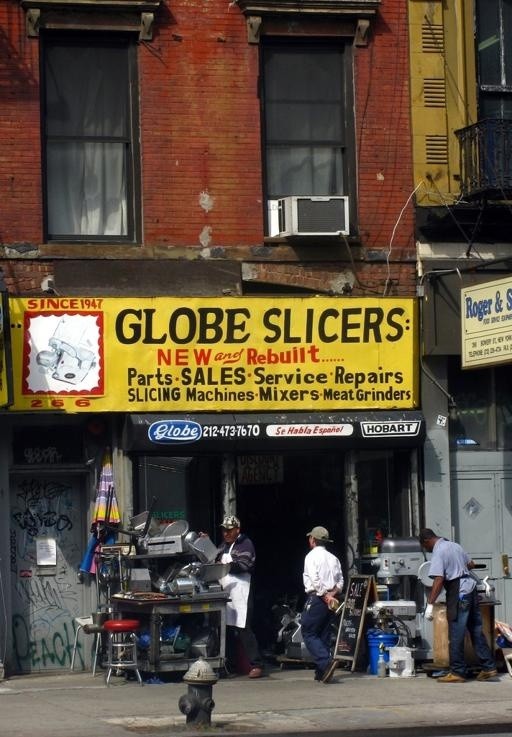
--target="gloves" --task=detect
[425,604,434,621]
[221,554,233,564]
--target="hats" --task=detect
[306,526,333,542]
[221,515,241,531]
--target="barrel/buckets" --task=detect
[368,633,399,675]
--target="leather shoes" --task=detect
[248,668,263,679]
[477,669,497,680]
[438,673,464,682]
[314,658,337,683]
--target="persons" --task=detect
[299,525,346,684]
[198,514,266,679]
[417,527,498,683]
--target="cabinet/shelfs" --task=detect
[433,600,502,673]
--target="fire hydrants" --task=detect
[178,655,219,729]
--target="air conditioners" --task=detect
[278,196,350,238]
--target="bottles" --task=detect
[377,654,386,677]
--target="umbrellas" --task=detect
[89,447,121,543]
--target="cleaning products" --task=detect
[377,641,385,677]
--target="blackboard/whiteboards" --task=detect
[333,574,371,660]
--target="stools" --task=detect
[101,619,143,687]
